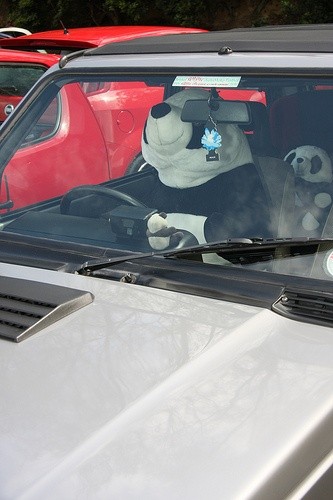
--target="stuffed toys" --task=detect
[131,88,272,271]
[281,145,332,242]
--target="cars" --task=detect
[0,24,332,216]
[0,26,48,54]
[0,23,333,500]
